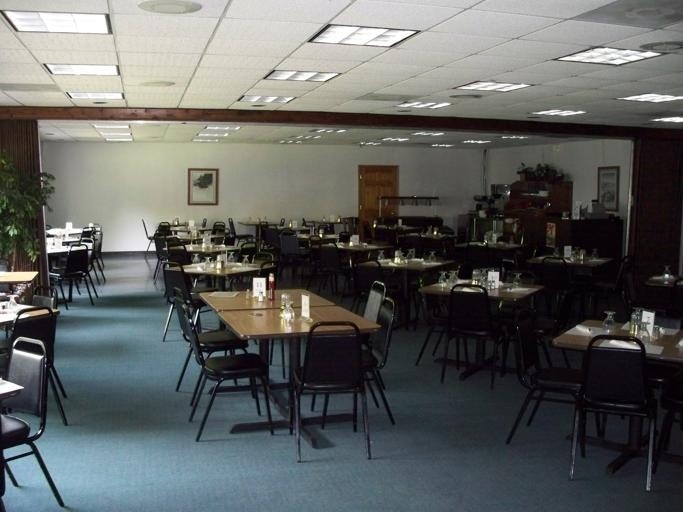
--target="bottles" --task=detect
[268,272,275,300]
[246,288,250,299]
[259,292,263,302]
[488,281,494,289]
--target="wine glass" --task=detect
[378,249,385,261]
[242,255,249,267]
[284,300,295,319]
[450,270,458,284]
[480,268,487,281]
[5,294,18,313]
[437,271,448,287]
[192,253,200,266]
[512,272,522,286]
[636,321,650,344]
[428,251,436,261]
[227,251,235,263]
[629,306,644,335]
[204,256,211,270]
[574,246,580,259]
[591,249,599,260]
[603,311,615,331]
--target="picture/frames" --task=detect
[596,166,619,212]
[187,168,218,205]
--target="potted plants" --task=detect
[516,162,561,181]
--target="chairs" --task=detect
[0,222,106,512]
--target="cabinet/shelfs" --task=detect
[510,180,572,217]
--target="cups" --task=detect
[472,268,480,285]
[280,292,290,310]
[577,252,584,259]
